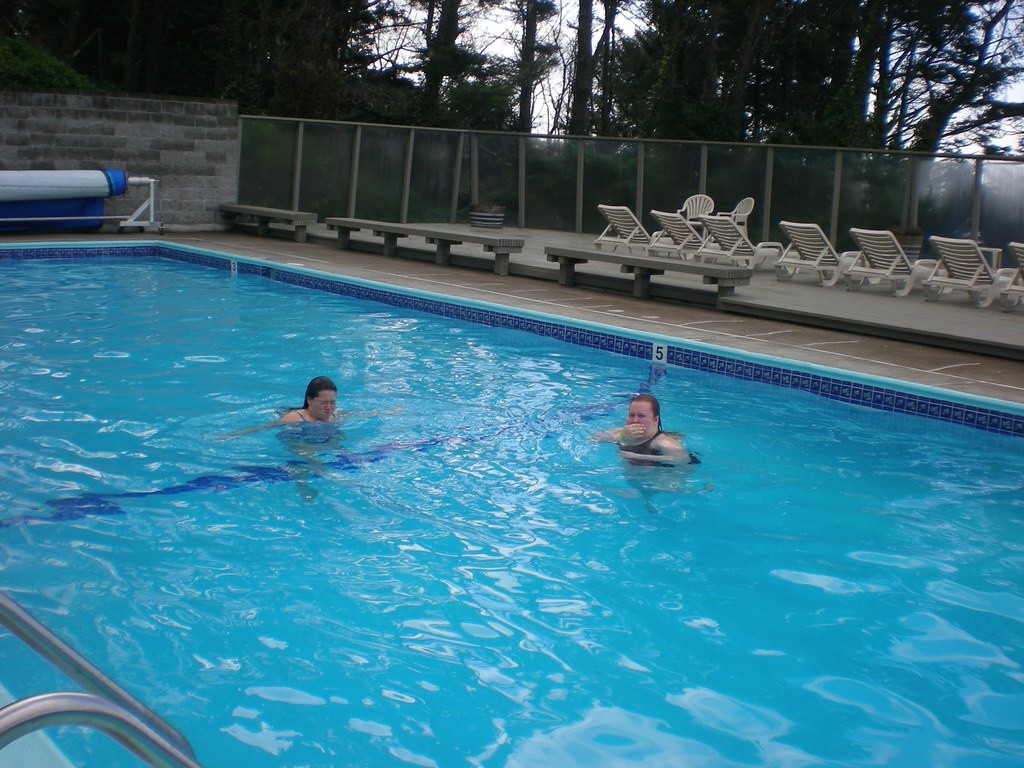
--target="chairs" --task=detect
[594,194,1024,314]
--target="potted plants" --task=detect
[468,203,505,227]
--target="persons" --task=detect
[215,375,405,505]
[590,394,715,513]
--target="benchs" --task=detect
[218,201,317,243]
[326,216,524,276]
[544,245,750,299]
